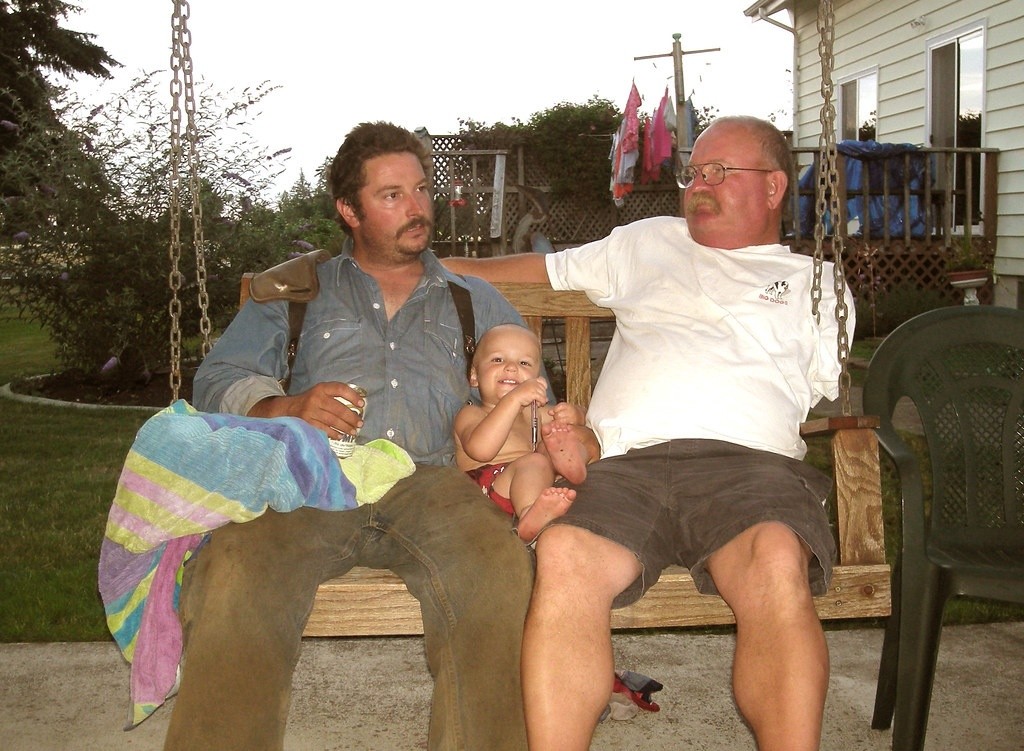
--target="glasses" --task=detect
[675,163,773,189]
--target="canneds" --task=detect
[325,382,368,460]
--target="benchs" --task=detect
[241,273,893,637]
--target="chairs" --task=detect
[862,302,1024,751]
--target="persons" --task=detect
[437,118,856,751]
[177,120,532,750]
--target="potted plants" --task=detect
[945,235,1000,284]
